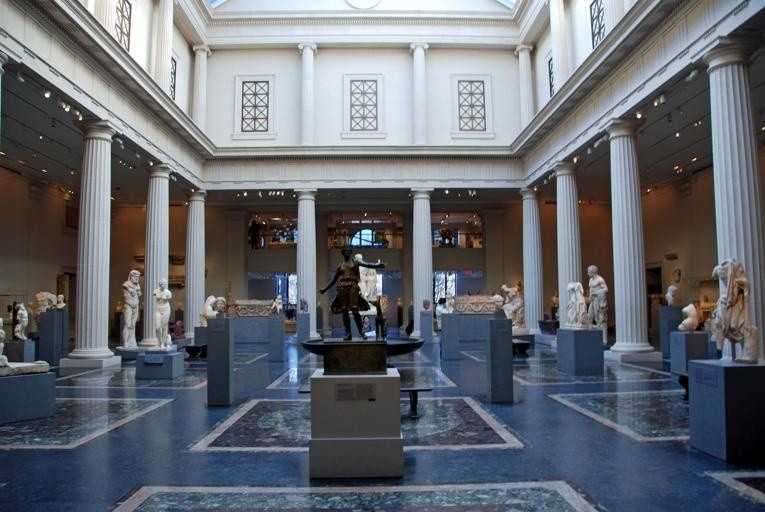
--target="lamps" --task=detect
[16,71,195,194]
[533,68,700,193]
[232,190,479,199]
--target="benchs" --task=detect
[297,382,432,419]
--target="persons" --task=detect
[665,284,678,307]
[152,278,173,349]
[201,294,219,321]
[361,314,372,334]
[574,281,588,326]
[319,245,385,341]
[354,251,371,301]
[586,264,611,349]
[271,294,283,315]
[551,291,559,322]
[215,296,227,311]
[121,270,144,348]
[435,299,453,361]
[500,284,523,324]
[566,282,577,325]
[55,294,66,309]
[368,269,379,297]
